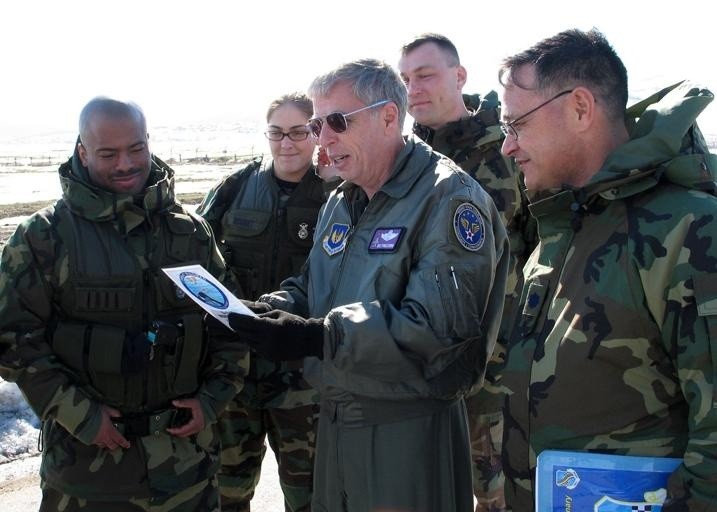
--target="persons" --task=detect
[467,28,717,512]
[400,34,522,223]
[195,92,343,512]
[229,59,509,512]
[0,93,253,512]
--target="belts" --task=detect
[109,407,193,443]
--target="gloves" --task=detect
[203,299,326,363]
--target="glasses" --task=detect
[264,130,310,142]
[498,90,598,142]
[305,99,391,139]
